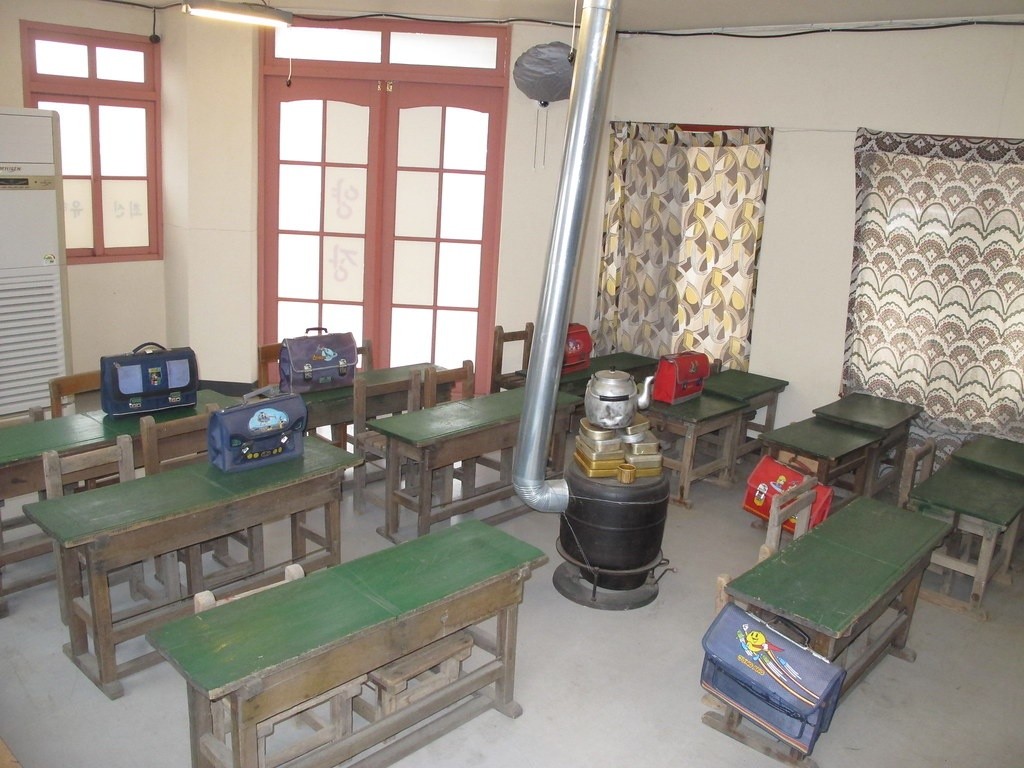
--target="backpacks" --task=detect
[650,349,710,407]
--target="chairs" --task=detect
[491,323,535,393]
[717,477,818,595]
[259,341,405,451]
[353,360,513,522]
[1,372,131,554]
[42,402,264,648]
[901,438,973,595]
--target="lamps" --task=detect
[182,0,293,28]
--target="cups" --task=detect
[616,464,637,484]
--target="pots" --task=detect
[559,461,671,569]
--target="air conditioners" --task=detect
[0,108,76,424]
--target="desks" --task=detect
[638,368,790,508]
[516,351,661,433]
[700,497,955,768]
[751,392,924,529]
[252,363,452,450]
[363,388,584,546]
[907,435,1024,623]
[0,389,247,616]
[144,518,550,768]
[22,436,363,700]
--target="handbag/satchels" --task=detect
[561,323,595,376]
[742,453,834,536]
[205,382,308,472]
[699,600,848,756]
[100,341,199,417]
[278,327,359,397]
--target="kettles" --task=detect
[583,366,655,430]
